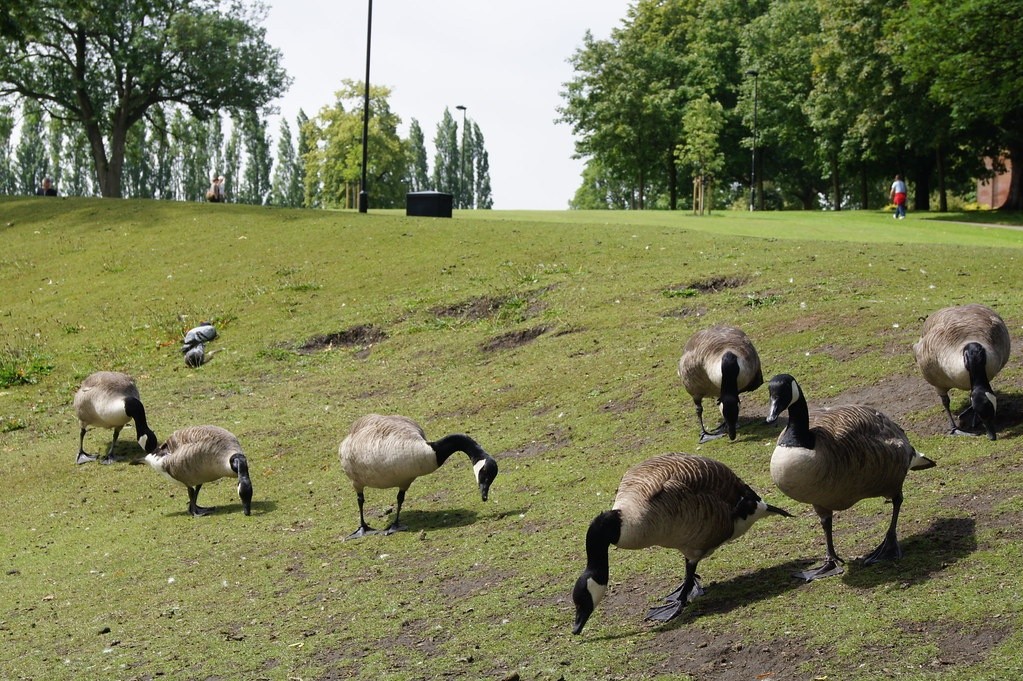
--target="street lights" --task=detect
[745,69,759,210]
[455,104,467,209]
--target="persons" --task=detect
[37,177,57,196]
[210,175,224,202]
[889,175,907,219]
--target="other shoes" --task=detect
[899,216,905,219]
[893,214,897,219]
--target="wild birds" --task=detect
[73,371,158,465]
[571,452,798,636]
[766,373,937,582]
[178,324,221,369]
[338,414,499,541]
[912,304,1010,441]
[678,325,765,444]
[129,424,253,516]
